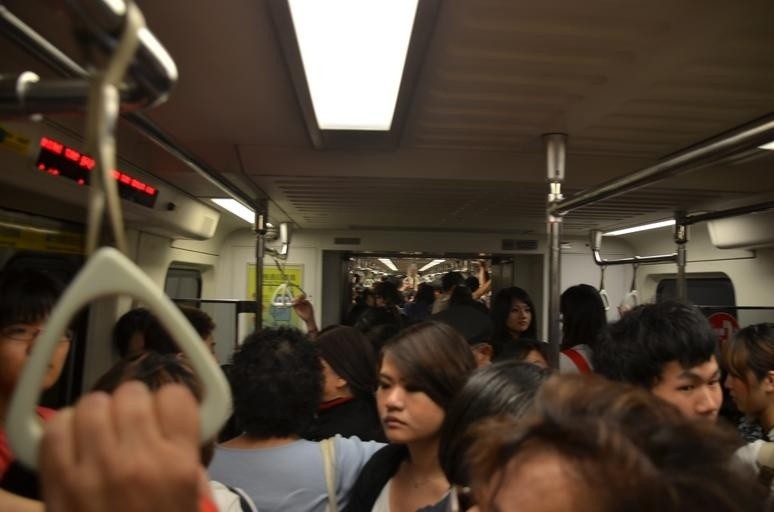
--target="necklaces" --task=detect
[406,474,438,488]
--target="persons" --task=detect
[724,322,774,444]
[509,338,555,371]
[293,296,378,441]
[92,303,216,392]
[492,286,538,348]
[411,360,549,512]
[431,271,492,315]
[440,284,489,334]
[36,381,205,511]
[465,261,485,293]
[342,287,375,328]
[204,326,389,512]
[336,319,479,512]
[464,372,706,512]
[381,275,404,292]
[352,274,361,290]
[0,250,91,512]
[399,284,436,323]
[590,300,723,427]
[557,283,607,377]
[374,281,403,329]
[402,270,419,292]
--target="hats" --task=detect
[320,325,376,397]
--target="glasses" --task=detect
[2,323,77,346]
